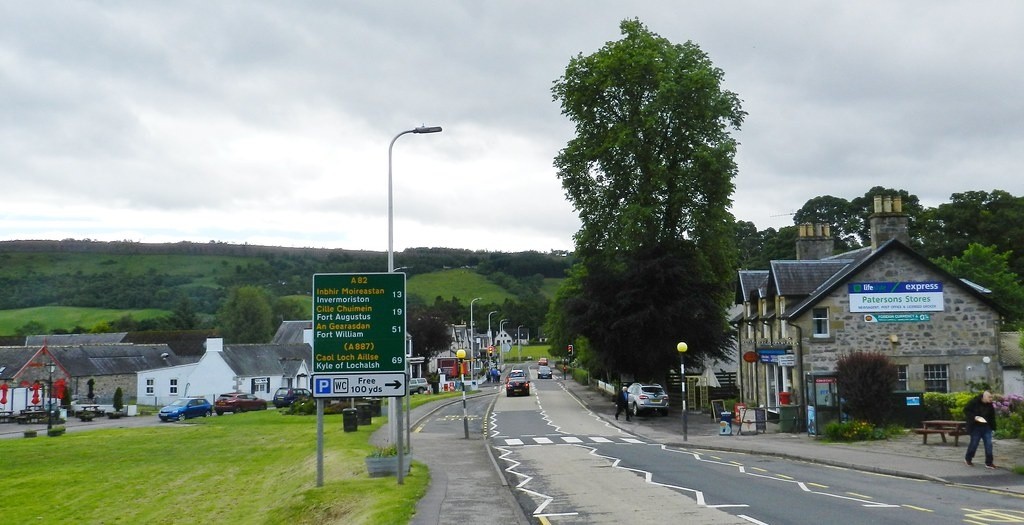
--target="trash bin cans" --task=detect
[778,405,799,432]
[357,403,371,426]
[368,399,381,417]
[343,408,357,432]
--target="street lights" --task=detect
[457,349,470,439]
[388,126,443,447]
[499,319,524,364]
[677,342,688,441]
[470,297,484,380]
[488,311,498,346]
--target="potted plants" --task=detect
[363,441,415,478]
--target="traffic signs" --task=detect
[312,371,407,398]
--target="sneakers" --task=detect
[985,463,998,469]
[964,459,974,467]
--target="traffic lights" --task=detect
[489,346,493,356]
[568,345,573,356]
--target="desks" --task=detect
[19,410,24,414]
[921,419,968,446]
[81,405,100,411]
[24,411,46,425]
[26,405,42,410]
[0,410,14,422]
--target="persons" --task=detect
[486,368,501,384]
[962,390,996,469]
[615,387,631,422]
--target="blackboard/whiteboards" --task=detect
[710,400,725,419]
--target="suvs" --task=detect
[627,383,669,417]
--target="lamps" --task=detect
[891,334,897,343]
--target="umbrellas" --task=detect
[56,378,65,405]
[32,383,40,412]
[0,383,8,424]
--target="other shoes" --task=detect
[614,414,618,421]
[626,419,632,423]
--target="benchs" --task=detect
[76,410,105,413]
[0,415,46,420]
[913,428,972,436]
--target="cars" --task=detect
[506,369,530,396]
[538,358,548,366]
[214,392,267,416]
[159,398,213,423]
[272,387,317,408]
[537,366,552,379]
